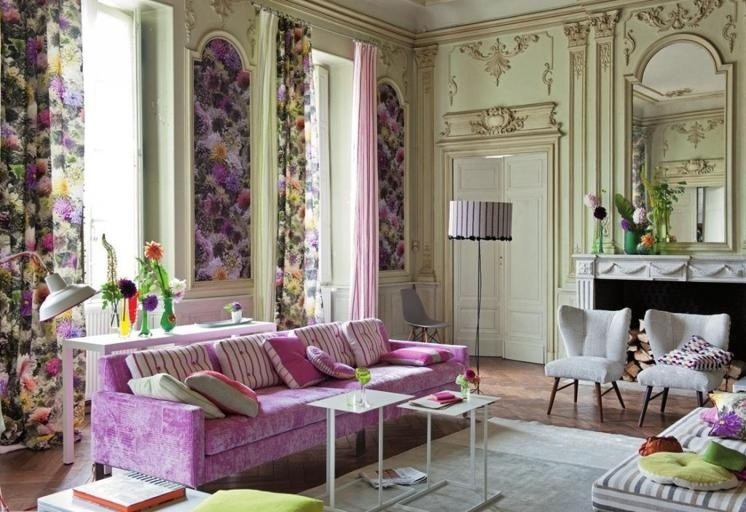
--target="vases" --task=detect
[118,298,131,337]
[646,210,669,255]
[138,306,154,337]
[635,243,648,254]
[107,301,121,337]
[623,229,644,255]
[231,310,242,324]
[460,385,469,400]
[590,221,603,254]
[159,299,177,332]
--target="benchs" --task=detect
[590,405,746,512]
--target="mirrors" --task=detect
[622,32,735,252]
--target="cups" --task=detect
[343,385,357,407]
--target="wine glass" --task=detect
[355,366,372,409]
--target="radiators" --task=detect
[82,304,158,399]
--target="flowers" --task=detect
[99,233,186,327]
[614,166,687,249]
[223,302,241,313]
[454,370,481,386]
[582,189,608,221]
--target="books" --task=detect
[429,393,461,404]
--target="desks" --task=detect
[304,388,417,512]
[33,481,213,511]
[60,319,277,466]
[392,389,503,512]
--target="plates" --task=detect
[194,317,253,328]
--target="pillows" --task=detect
[707,392,746,441]
[701,406,718,425]
[341,317,391,368]
[383,345,453,368]
[211,332,284,391]
[290,321,356,369]
[261,336,326,391]
[122,342,214,385]
[304,345,358,381]
[657,334,735,371]
[183,368,260,420]
[185,489,324,512]
[124,373,226,420]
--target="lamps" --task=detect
[0,251,97,323]
[446,200,512,396]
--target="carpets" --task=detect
[296,415,647,512]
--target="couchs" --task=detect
[90,317,470,493]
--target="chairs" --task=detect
[543,305,632,424]
[399,288,450,343]
[635,309,731,427]
